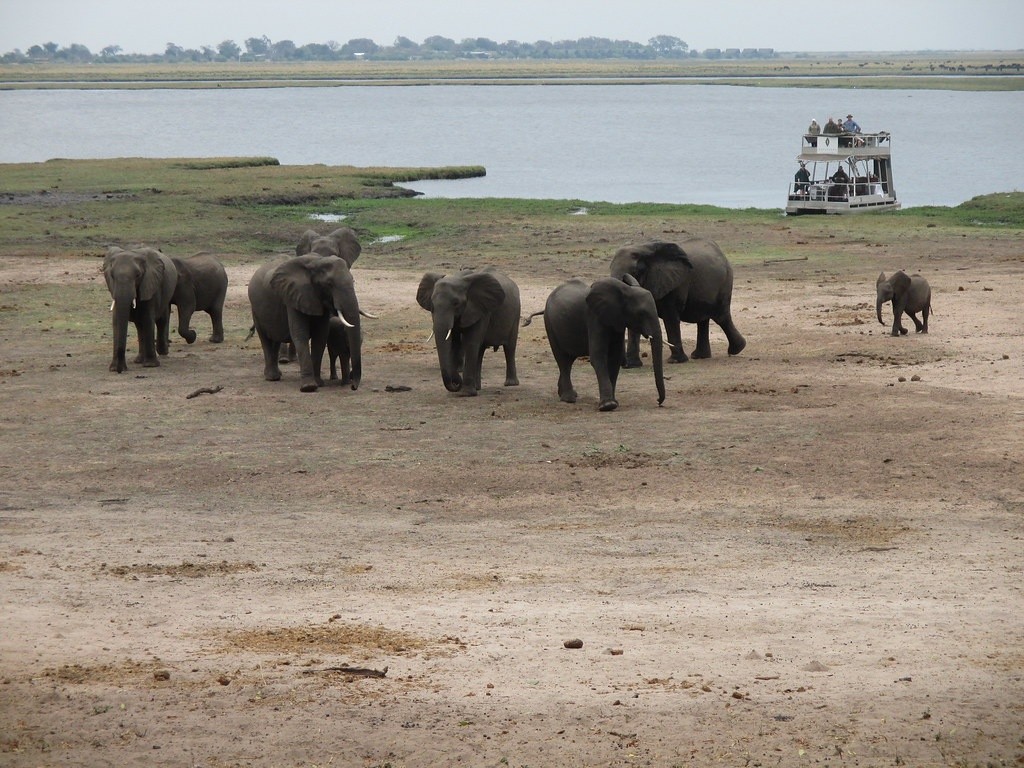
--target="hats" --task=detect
[847,114,853,118]
[812,119,815,122]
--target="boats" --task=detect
[785,133,902,215]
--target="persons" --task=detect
[828,165,850,202]
[808,114,860,148]
[794,163,810,195]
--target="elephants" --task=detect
[874,270,934,337]
[100,224,748,412]
